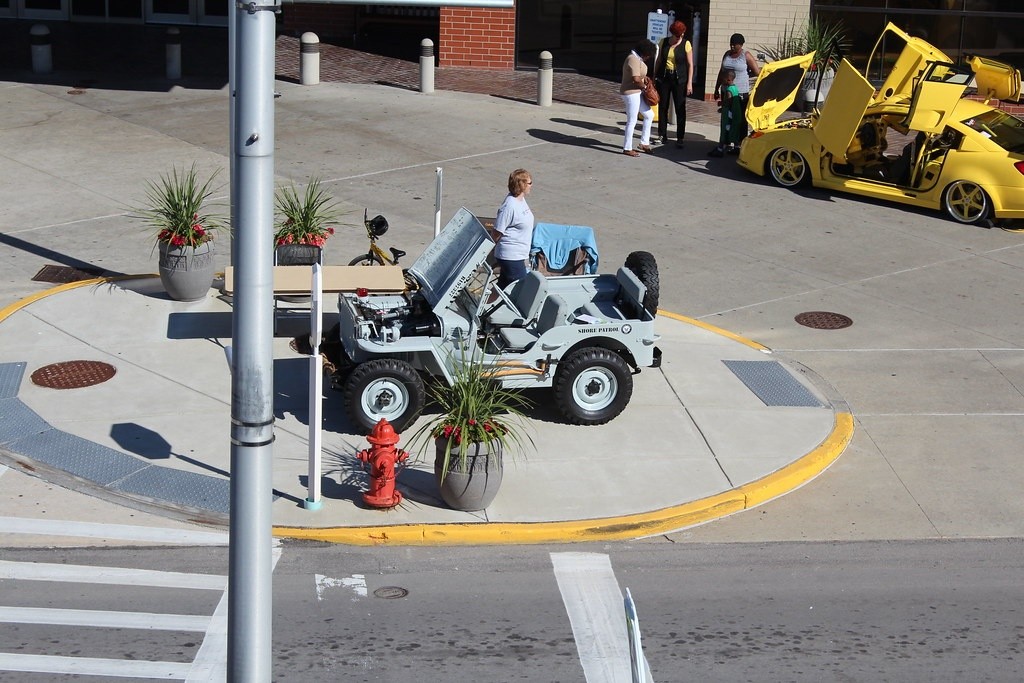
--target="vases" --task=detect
[274,244,323,304]
[158,240,215,303]
[796,68,836,113]
[434,436,504,513]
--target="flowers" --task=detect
[273,168,361,249]
[120,156,235,265]
[748,10,853,92]
[395,323,539,488]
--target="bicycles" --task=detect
[347,209,423,301]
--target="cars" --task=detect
[735,25,1024,229]
[331,202,664,434]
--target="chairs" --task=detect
[484,270,549,331]
[499,293,568,350]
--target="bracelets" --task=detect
[642,86,646,90]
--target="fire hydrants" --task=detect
[356,417,410,509]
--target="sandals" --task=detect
[623,151,640,157]
[637,144,655,155]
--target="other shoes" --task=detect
[717,143,741,154]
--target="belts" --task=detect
[665,69,675,74]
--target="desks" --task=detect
[223,264,406,335]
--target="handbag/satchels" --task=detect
[642,80,659,107]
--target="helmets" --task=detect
[369,215,388,236]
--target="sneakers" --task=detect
[675,140,685,149]
[653,136,668,145]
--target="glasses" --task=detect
[527,181,532,185]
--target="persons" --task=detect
[653,21,693,143]
[486,168,535,304]
[708,34,759,157]
[621,39,656,156]
